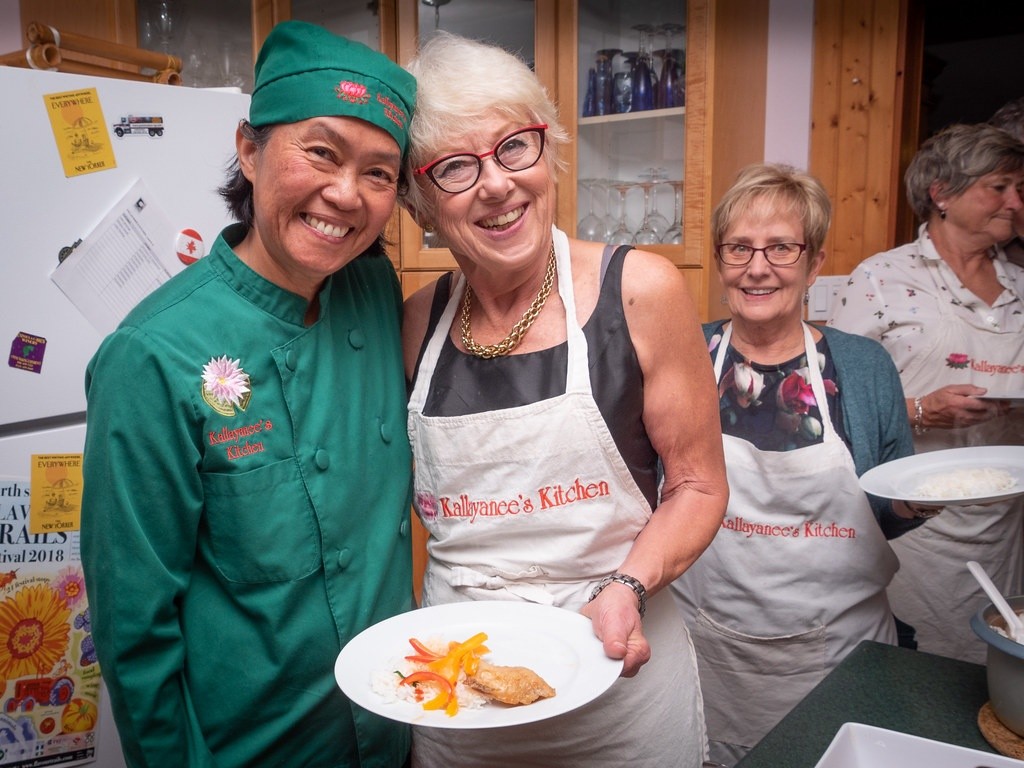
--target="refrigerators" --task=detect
[1,64,253,767]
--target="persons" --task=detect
[390,31,728,767]
[656,161,947,768]
[76,20,412,767]
[820,121,1024,663]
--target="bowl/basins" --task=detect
[970,594,1024,738]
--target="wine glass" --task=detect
[577,163,682,244]
[597,24,685,116]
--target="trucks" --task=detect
[112,114,163,138]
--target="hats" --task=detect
[247,18,417,159]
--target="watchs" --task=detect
[902,499,944,519]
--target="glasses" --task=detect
[413,122,547,195]
[717,242,808,265]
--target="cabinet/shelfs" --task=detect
[252,1,772,328]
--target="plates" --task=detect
[814,722,1024,768]
[858,445,1024,507]
[334,600,625,729]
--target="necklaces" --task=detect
[458,238,558,359]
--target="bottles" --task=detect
[586,60,611,118]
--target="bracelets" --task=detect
[586,573,647,620]
[913,396,932,437]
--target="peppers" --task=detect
[401,632,488,715]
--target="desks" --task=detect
[733,639,1024,767]
